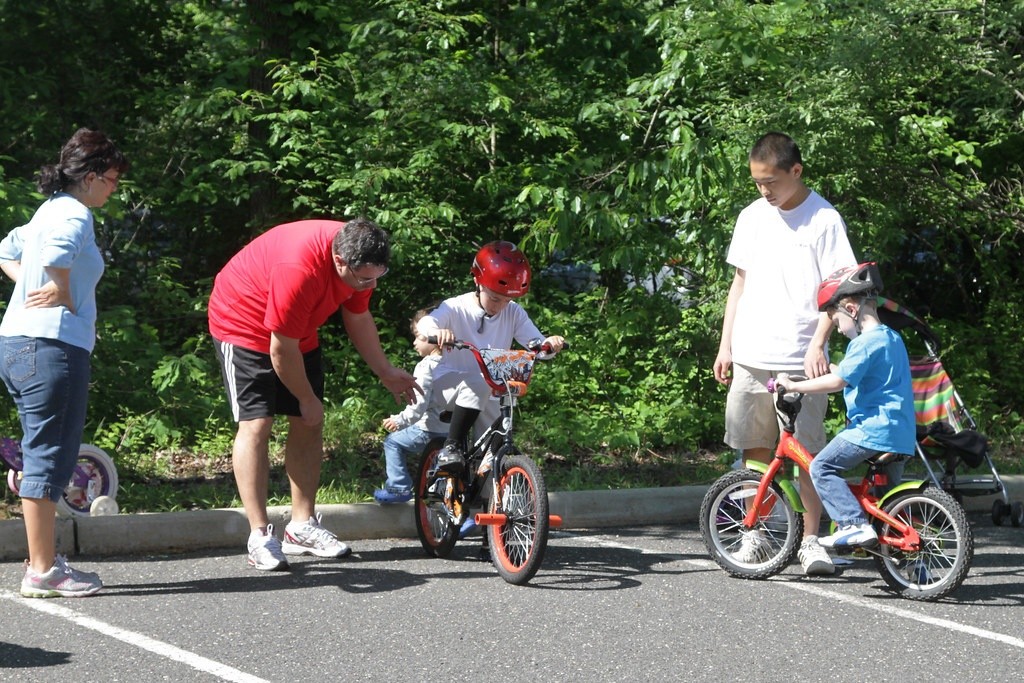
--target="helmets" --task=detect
[470,239,532,296]
[817,261,887,312]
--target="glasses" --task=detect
[98,173,119,187]
[342,258,389,284]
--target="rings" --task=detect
[401,391,406,397]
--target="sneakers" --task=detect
[904,563,930,584]
[730,529,772,565]
[478,541,525,565]
[437,441,467,473]
[816,523,878,549]
[19,553,103,597]
[796,533,836,575]
[281,511,348,558]
[247,522,290,571]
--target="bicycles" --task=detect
[0,427,120,519]
[697,375,976,599]
[412,335,571,585]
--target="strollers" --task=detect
[871,296,1024,531]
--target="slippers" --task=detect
[374,489,413,503]
[443,517,477,540]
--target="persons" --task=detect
[0,128,130,597]
[375,307,455,505]
[773,264,934,582]
[713,130,859,574]
[417,243,563,566]
[208,219,425,571]
